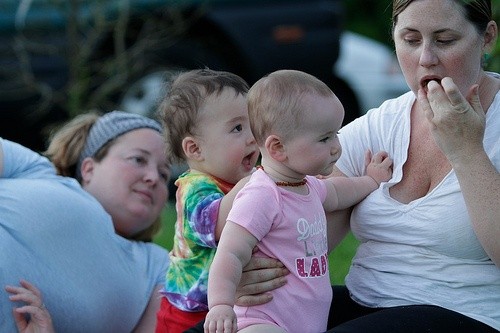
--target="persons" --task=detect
[204,68,393,333]
[155,69,261,333]
[237,0,500,333]
[0,109,174,333]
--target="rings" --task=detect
[40,302,45,310]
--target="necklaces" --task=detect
[257,163,309,187]
